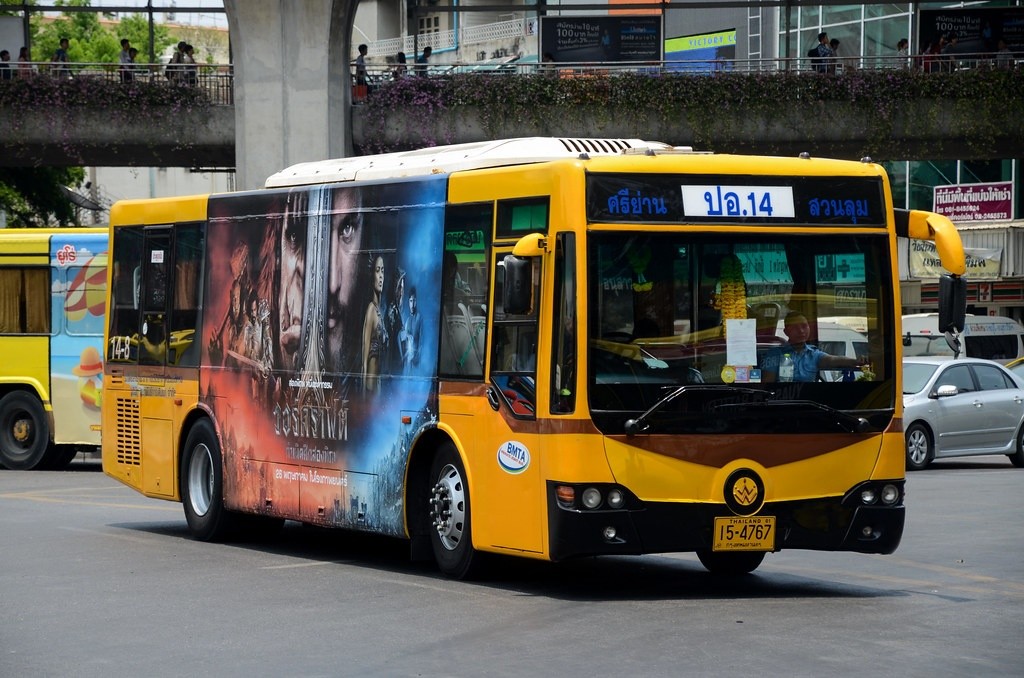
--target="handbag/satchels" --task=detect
[166,52,180,80]
[47,52,60,78]
[20,61,32,79]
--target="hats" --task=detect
[423,47,432,51]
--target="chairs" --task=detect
[751,303,781,343]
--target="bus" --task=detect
[96,136,967,580]
[0,227,109,471]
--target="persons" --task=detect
[601,295,635,374]
[897,39,908,63]
[0,50,11,80]
[419,47,432,73]
[356,44,373,94]
[119,39,137,82]
[396,52,407,73]
[996,40,1013,58]
[762,311,866,382]
[19,47,32,83]
[921,31,958,72]
[444,252,472,302]
[52,38,74,76]
[818,33,839,73]
[207,188,424,398]
[171,42,198,86]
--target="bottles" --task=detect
[779,354,794,382]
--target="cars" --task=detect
[903,357,1024,469]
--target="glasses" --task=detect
[396,55,400,58]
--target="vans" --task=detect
[774,312,1024,381]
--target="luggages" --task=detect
[353,70,368,103]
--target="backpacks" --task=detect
[924,47,940,72]
[808,45,829,70]
